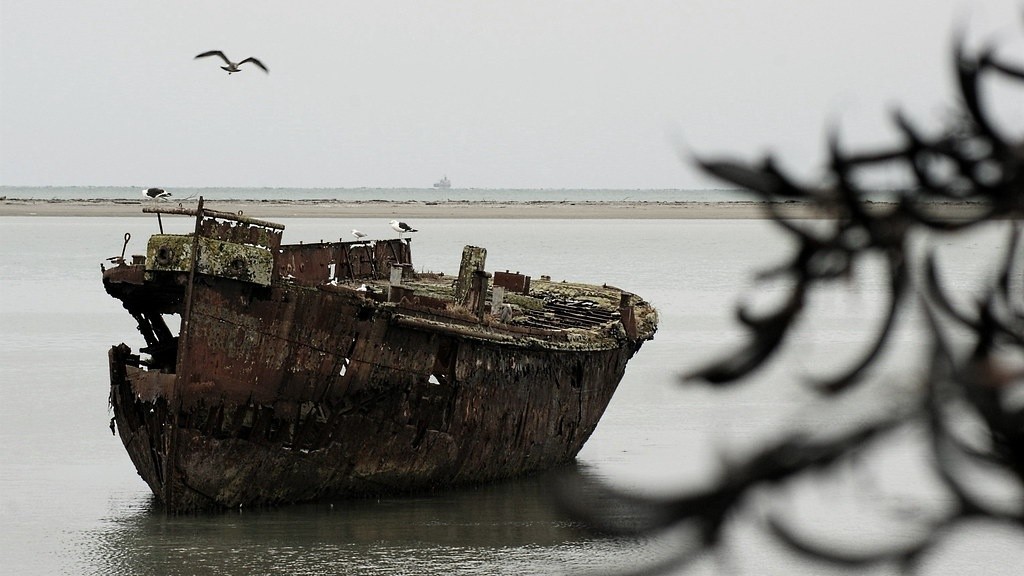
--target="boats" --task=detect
[99,196,662,519]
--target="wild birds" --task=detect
[193,50,269,76]
[140,187,172,206]
[389,219,419,239]
[351,230,368,240]
